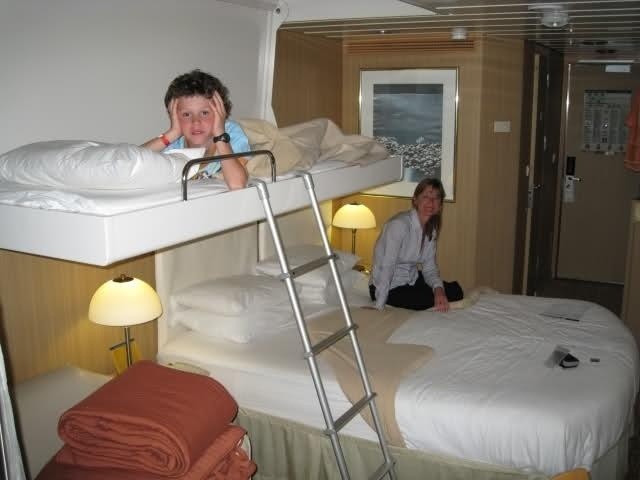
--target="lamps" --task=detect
[332,199,376,256]
[88,272,164,368]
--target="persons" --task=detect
[137,68,251,192]
[360,179,463,313]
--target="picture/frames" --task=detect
[357,66,459,204]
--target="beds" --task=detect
[0,121,406,268]
[161,247,639,480]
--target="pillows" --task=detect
[1,139,193,200]
[13,361,116,479]
[171,246,362,345]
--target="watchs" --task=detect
[213,133,230,143]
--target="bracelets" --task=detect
[159,134,171,147]
